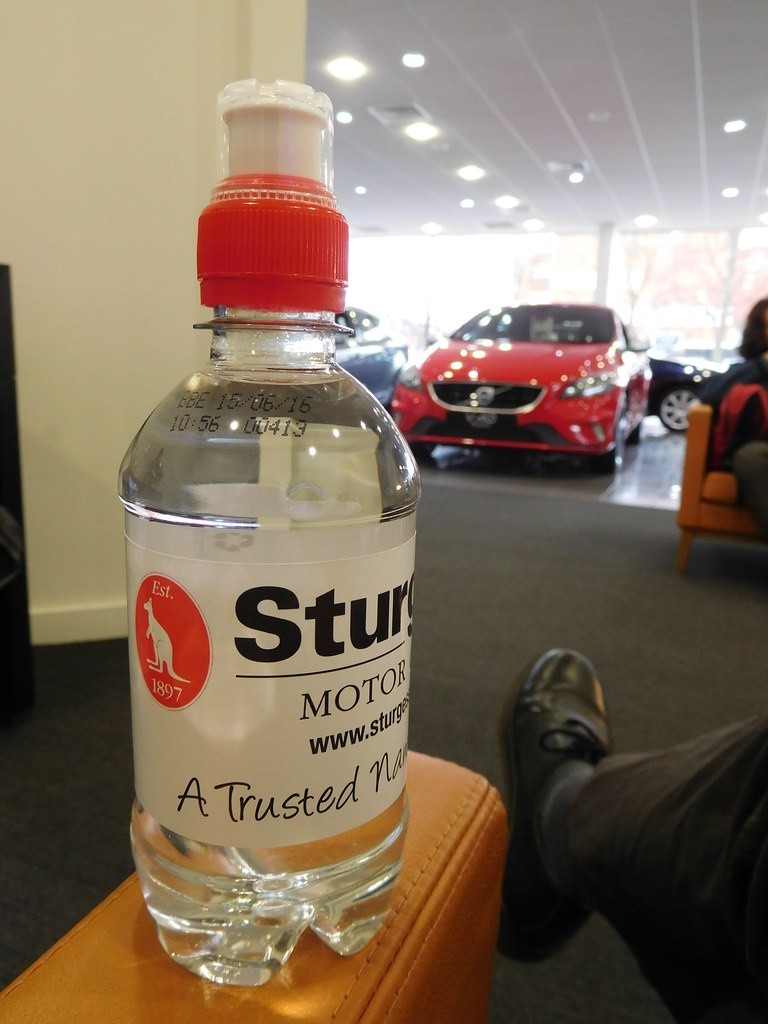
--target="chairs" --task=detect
[678,403,767,574]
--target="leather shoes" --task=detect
[496,646,611,965]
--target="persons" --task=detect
[500,648,768,1024]
[710,299,768,543]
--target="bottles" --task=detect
[117,76,423,988]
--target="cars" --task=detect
[333,326,414,411]
[644,347,730,434]
[384,300,652,477]
